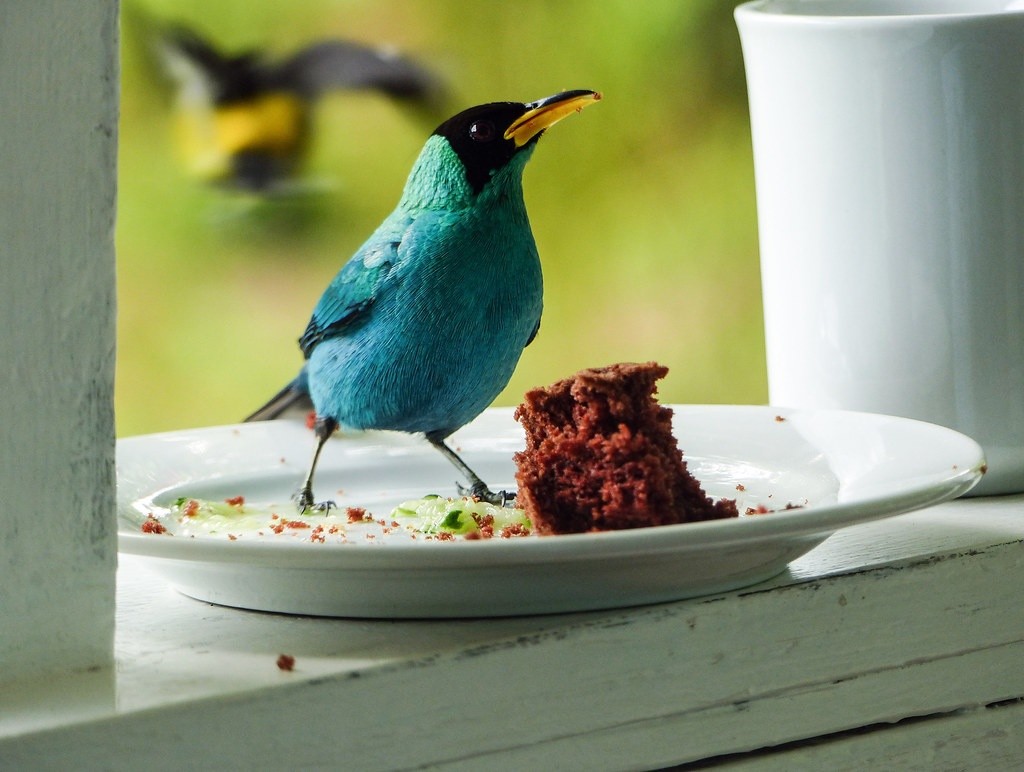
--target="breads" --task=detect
[510,362,740,535]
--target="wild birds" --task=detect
[238,87,601,516]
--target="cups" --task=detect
[732,2,1024,499]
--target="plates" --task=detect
[116,406,988,619]
